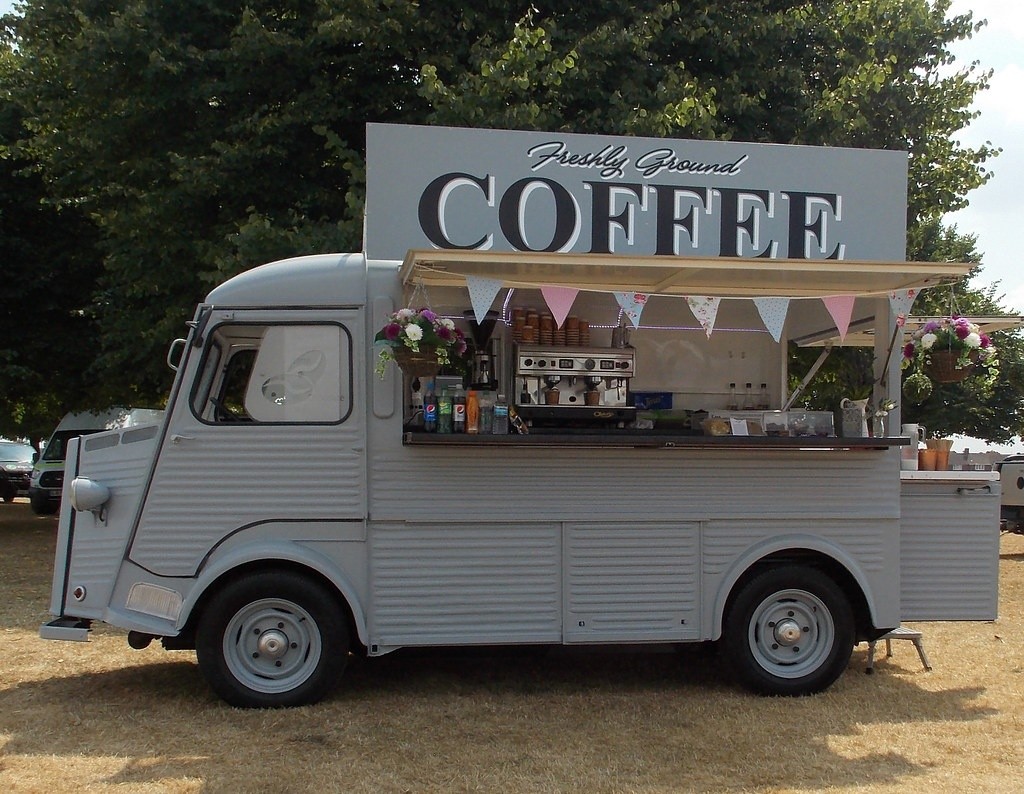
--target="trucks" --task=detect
[38,120,1024,709]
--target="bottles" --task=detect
[743,383,754,410]
[479,391,491,434]
[423,384,438,432]
[509,406,529,435]
[842,402,862,437]
[758,383,769,410]
[466,390,479,433]
[492,394,508,434]
[521,383,530,404]
[452,383,466,433]
[727,383,738,410]
[438,384,452,434]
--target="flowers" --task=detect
[874,399,897,416]
[374,309,469,378]
[904,314,992,359]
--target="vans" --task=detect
[0,442,39,502]
[29,406,166,516]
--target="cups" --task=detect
[512,306,539,346]
[539,312,553,347]
[545,389,560,405]
[554,318,565,347]
[579,319,590,347]
[919,449,937,471]
[566,317,579,347]
[584,391,600,406]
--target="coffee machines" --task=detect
[463,309,499,391]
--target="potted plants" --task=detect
[830,352,880,437]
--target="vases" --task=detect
[873,416,885,438]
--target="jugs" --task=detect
[901,424,926,470]
[612,322,631,349]
[840,398,870,437]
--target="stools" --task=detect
[866,628,933,674]
